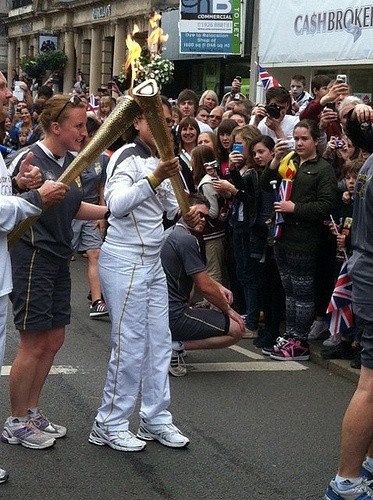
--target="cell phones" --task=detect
[236,76,241,82]
[337,75,347,84]
[233,144,243,154]
[326,102,336,112]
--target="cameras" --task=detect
[280,140,297,150]
[263,103,282,119]
[361,121,373,130]
[334,140,345,149]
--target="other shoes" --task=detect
[350,343,363,369]
[242,325,260,338]
[71,254,74,261]
[252,328,282,349]
[320,342,353,359]
[82,250,88,257]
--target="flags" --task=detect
[257,63,281,91]
[326,262,354,315]
[328,302,356,334]
[86,93,99,114]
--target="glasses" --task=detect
[199,211,211,222]
[136,115,176,128]
[54,94,81,122]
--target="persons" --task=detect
[0,68,373,500]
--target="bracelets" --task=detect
[146,172,161,189]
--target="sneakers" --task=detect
[168,349,189,377]
[323,334,341,345]
[26,406,67,439]
[324,477,373,500]
[261,336,295,354]
[270,340,310,360]
[88,418,146,451]
[0,469,9,483]
[308,319,329,339]
[360,458,373,481]
[89,300,108,317]
[137,418,190,449]
[0,417,56,450]
